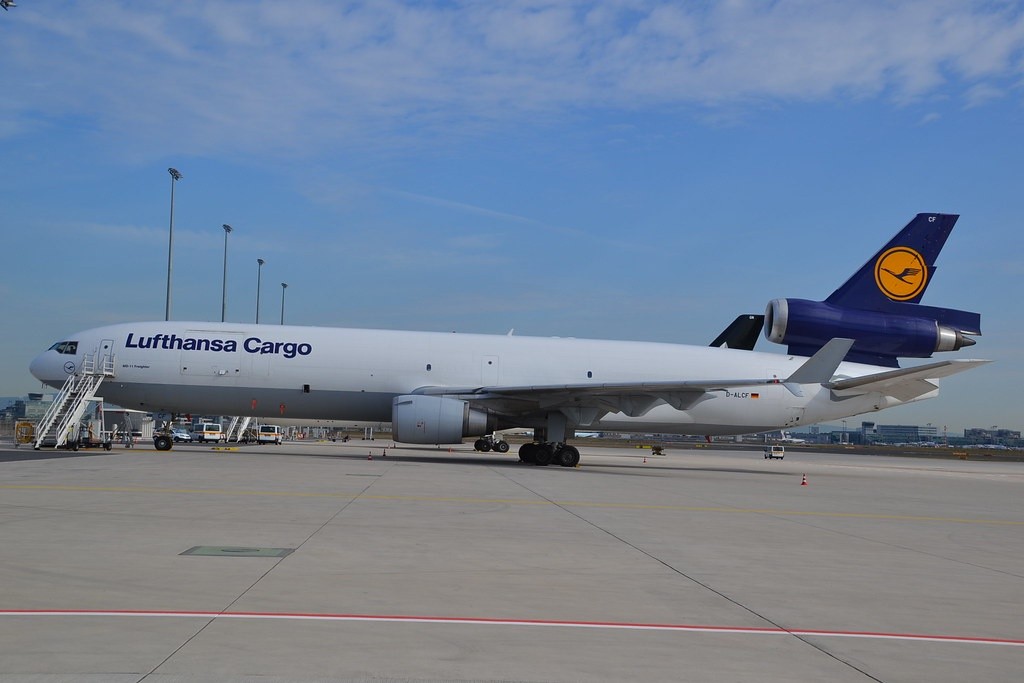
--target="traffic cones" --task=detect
[383,449,387,457]
[801,474,807,485]
[368,451,372,460]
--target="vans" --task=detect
[763,446,785,460]
[257,425,284,445]
[190,423,219,443]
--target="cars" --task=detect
[152,428,191,443]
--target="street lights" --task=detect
[281,282,288,325]
[165,167,182,321]
[221,223,233,322]
[256,258,265,324]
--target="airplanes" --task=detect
[30,212,1000,468]
